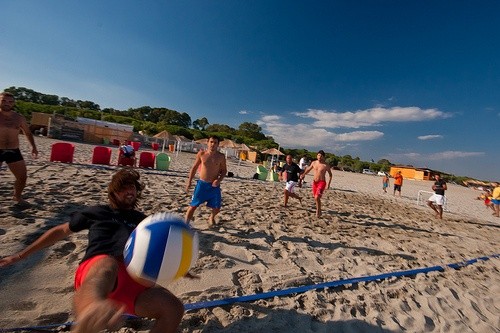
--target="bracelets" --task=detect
[17,253,23,259]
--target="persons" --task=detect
[299,155,307,185]
[482,182,500,217]
[183,136,227,228]
[275,155,306,208]
[300,150,333,218]
[427,173,447,219]
[392,170,403,197]
[0,91,39,204]
[0,168,186,333]
[382,174,390,193]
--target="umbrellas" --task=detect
[463,178,494,188]
[153,130,285,164]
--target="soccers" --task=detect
[123,212,198,288]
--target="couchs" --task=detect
[270,167,281,181]
[156,153,171,170]
[92,146,112,165]
[50,142,75,164]
[118,147,137,168]
[139,152,156,169]
[257,165,268,180]
